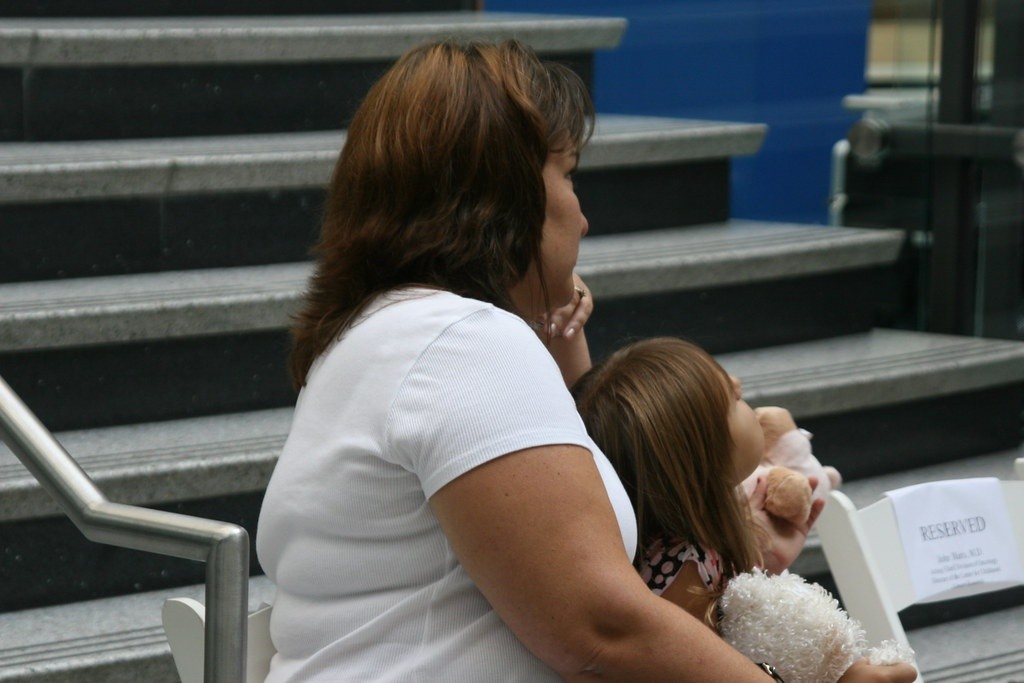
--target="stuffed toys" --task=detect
[720,568,916,683]
[735,403,842,522]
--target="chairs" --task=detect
[816,456,1024,683]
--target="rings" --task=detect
[574,286,587,299]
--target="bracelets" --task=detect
[754,661,786,683]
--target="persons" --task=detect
[570,338,824,639]
[255,38,917,683]
